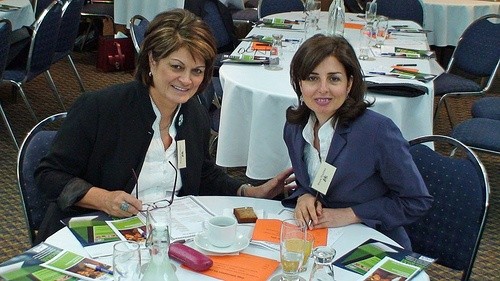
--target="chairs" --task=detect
[0,0,117,149]
[259,0,307,24]
[403,13,500,127]
[184,0,259,153]
[448,95,500,156]
[405,135,491,281]
[16,113,69,248]
[370,0,425,28]
[130,15,151,53]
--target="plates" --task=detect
[194,231,249,253]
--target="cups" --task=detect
[203,217,236,247]
[305,15,318,40]
[359,25,372,58]
[366,2,377,27]
[280,219,307,281]
[306,0,321,29]
[375,16,388,47]
[113,241,141,281]
[270,34,283,68]
[285,231,313,271]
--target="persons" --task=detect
[32,8,297,248]
[125,232,144,242]
[281,34,435,252]
[370,272,391,281]
[75,266,106,279]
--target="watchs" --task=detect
[241,183,254,197]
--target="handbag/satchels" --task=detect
[95,33,136,73]
[5,19,38,70]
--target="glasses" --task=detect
[132,161,177,212]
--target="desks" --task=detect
[114,0,185,29]
[216,10,445,190]
[0,0,35,32]
[422,1,500,48]
[46,196,430,281]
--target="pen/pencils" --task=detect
[135,223,149,245]
[394,63,418,67]
[306,191,320,228]
[82,263,114,276]
[172,236,195,247]
[282,37,299,43]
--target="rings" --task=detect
[120,202,131,211]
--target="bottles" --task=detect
[309,246,336,281]
[327,0,345,38]
[144,204,177,281]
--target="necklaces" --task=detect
[164,119,171,130]
